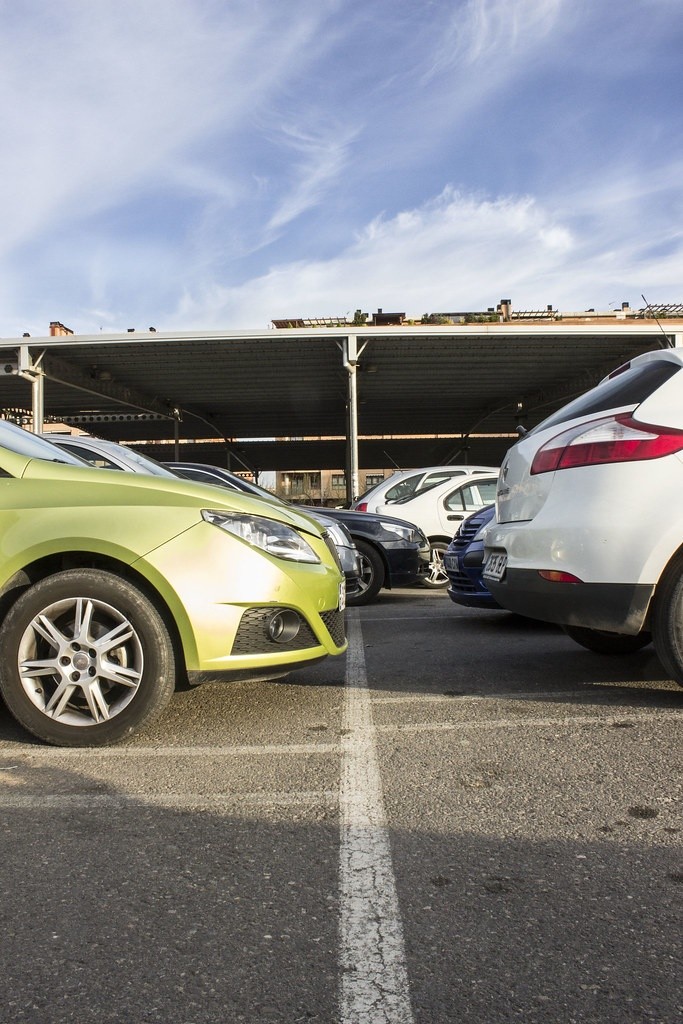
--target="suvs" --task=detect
[481,347,683,687]
[41,431,511,616]
[0,417,350,748]
